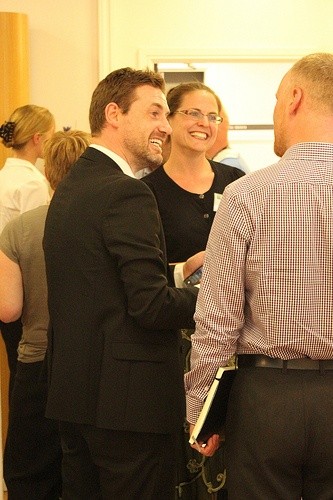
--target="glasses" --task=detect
[172,109,223,125]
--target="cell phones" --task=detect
[182,264,203,288]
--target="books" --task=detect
[189,366,238,444]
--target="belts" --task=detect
[233,354,333,372]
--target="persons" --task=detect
[0,104,92,500]
[183,53,333,500]
[139,80,259,500]
[42,66,204,499]
